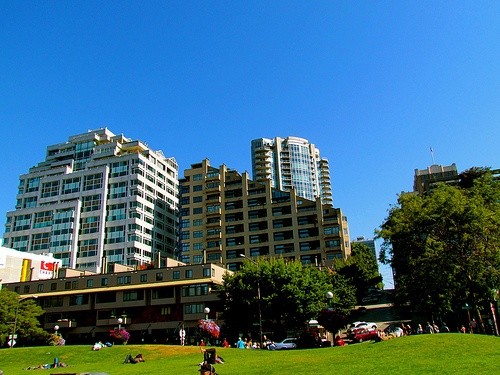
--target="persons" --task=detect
[39,357,68,368]
[193,332,275,350]
[122,353,145,364]
[92,340,106,351]
[334,329,345,345]
[197,346,225,366]
[374,317,494,342]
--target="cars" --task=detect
[347,322,377,334]
[275,338,298,350]
[347,328,378,343]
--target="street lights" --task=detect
[54,325,59,336]
[240,254,264,349]
[117,318,123,330]
[204,307,210,320]
[326,292,334,308]
